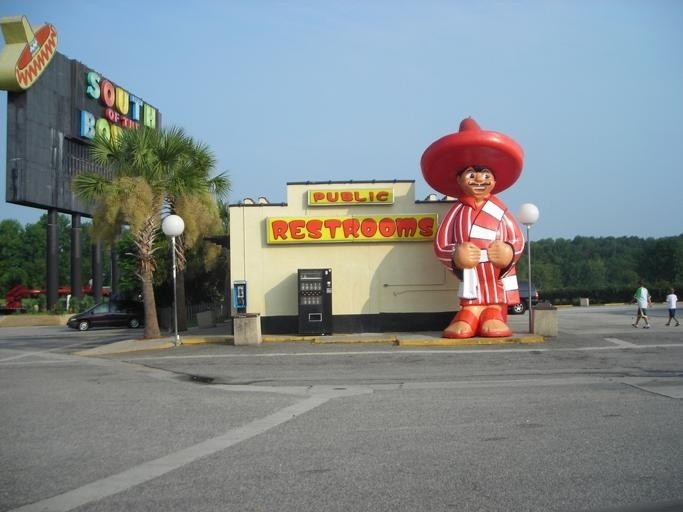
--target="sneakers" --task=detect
[665,323,680,326]
[632,324,650,328]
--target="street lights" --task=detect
[518,202,539,336]
[162,215,185,347]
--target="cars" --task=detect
[511,281,539,314]
[66,301,144,331]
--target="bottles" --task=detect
[301,282,322,304]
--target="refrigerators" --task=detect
[298,268,332,336]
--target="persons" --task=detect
[664,287,681,327]
[419,118,525,340]
[626,280,654,329]
